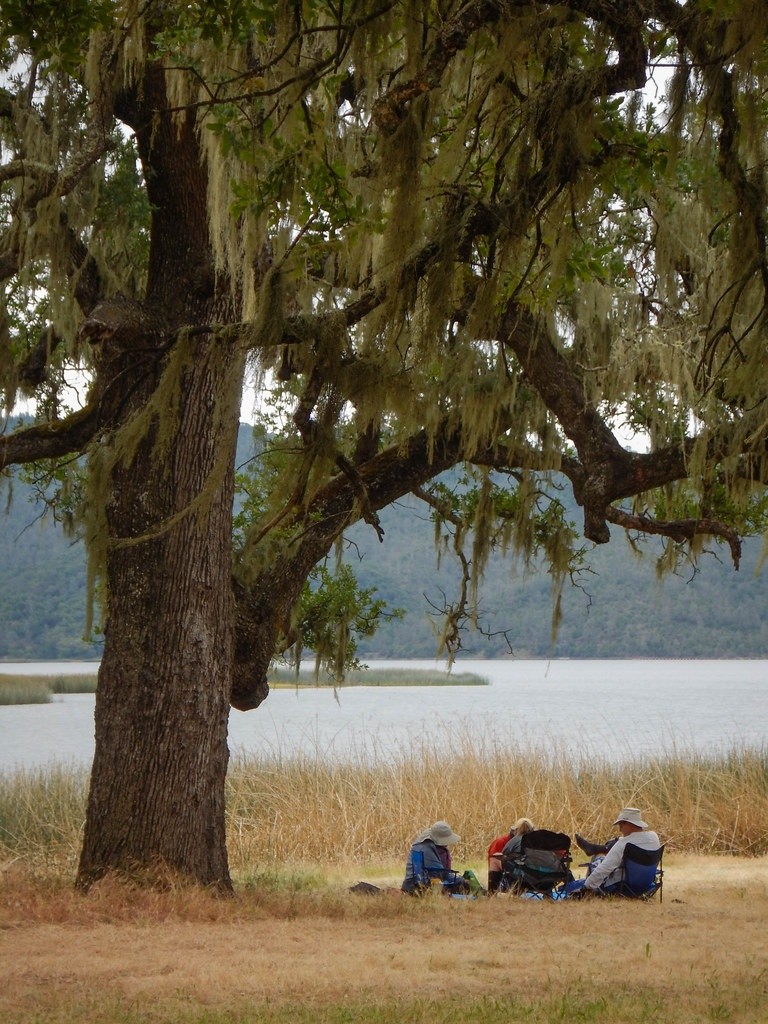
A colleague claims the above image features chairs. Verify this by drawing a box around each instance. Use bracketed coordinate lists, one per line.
[411, 849, 463, 898]
[490, 829, 572, 901]
[578, 842, 667, 904]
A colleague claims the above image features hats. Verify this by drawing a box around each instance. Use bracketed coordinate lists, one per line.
[417, 820, 461, 846]
[613, 808, 649, 828]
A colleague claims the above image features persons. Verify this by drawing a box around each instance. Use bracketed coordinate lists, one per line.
[502, 817, 553, 899]
[486, 822, 517, 894]
[575, 809, 659, 891]
[404, 819, 514, 900]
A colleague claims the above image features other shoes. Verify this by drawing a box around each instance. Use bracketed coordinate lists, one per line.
[575, 834, 594, 856]
